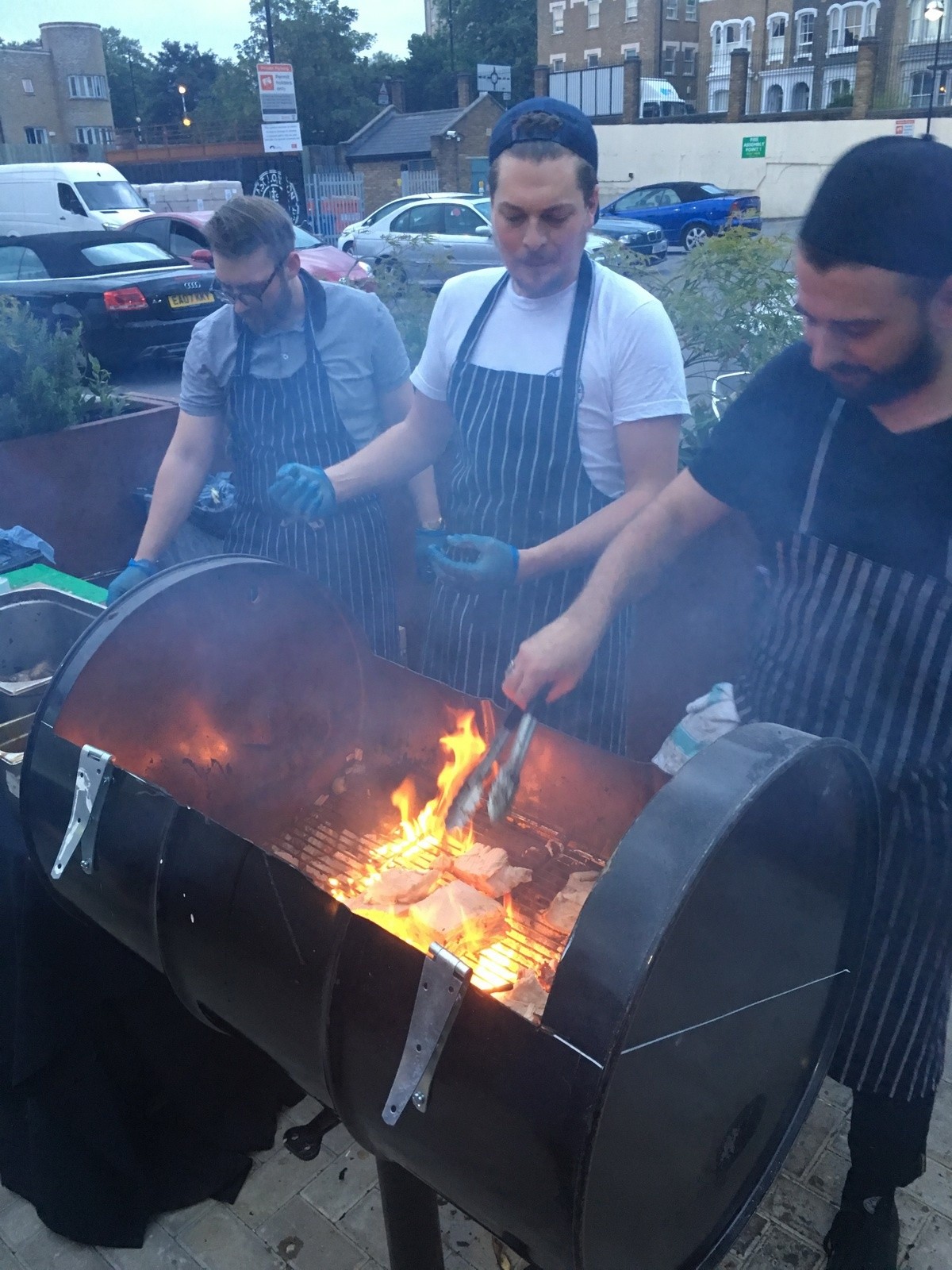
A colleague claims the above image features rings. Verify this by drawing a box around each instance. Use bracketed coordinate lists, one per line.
[511, 660, 514, 668]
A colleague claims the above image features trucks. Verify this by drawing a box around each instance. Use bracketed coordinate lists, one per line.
[638, 78, 687, 119]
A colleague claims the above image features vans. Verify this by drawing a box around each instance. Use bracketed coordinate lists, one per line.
[0, 162, 156, 236]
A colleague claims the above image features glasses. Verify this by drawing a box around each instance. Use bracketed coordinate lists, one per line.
[209, 259, 286, 309]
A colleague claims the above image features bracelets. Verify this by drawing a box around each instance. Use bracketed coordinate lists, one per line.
[419, 518, 444, 530]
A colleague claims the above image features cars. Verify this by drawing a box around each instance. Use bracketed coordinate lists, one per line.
[337, 192, 624, 299]
[116, 210, 378, 296]
[587, 214, 670, 267]
[597, 181, 763, 253]
[0, 233, 227, 387]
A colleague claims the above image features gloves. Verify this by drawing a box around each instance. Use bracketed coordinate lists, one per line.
[103, 558, 159, 607]
[271, 462, 339, 524]
[427, 532, 520, 594]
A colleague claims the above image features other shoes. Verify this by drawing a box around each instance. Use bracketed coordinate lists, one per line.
[823, 1168, 902, 1269]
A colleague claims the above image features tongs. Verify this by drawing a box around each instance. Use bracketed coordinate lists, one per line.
[443, 682, 554, 827]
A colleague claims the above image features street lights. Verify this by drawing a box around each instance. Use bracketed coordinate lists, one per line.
[136, 117, 142, 144]
[924, 0, 945, 137]
[178, 86, 187, 118]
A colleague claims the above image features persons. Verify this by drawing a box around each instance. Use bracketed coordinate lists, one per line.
[106, 193, 448, 665]
[501, 135, 952, 1270]
[268, 97, 691, 756]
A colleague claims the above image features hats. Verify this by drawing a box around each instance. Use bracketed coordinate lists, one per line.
[487, 96, 600, 224]
[800, 133, 952, 282]
